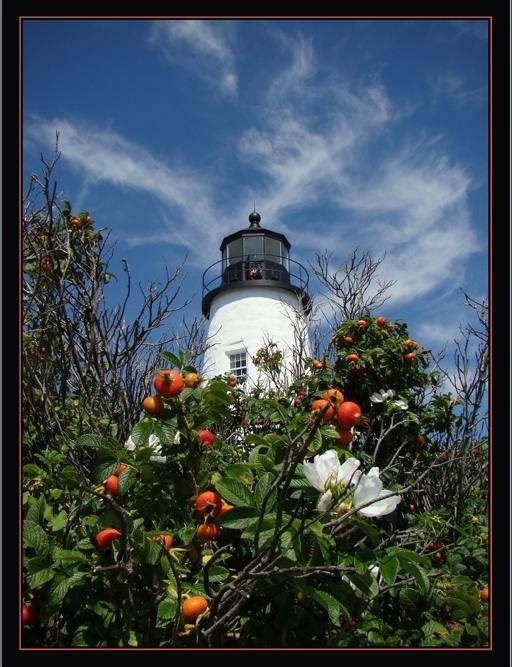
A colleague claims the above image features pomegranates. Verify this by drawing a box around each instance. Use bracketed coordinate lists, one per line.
[94, 317, 444, 620]
[23, 604, 30, 624]
[481, 588, 488, 602]
